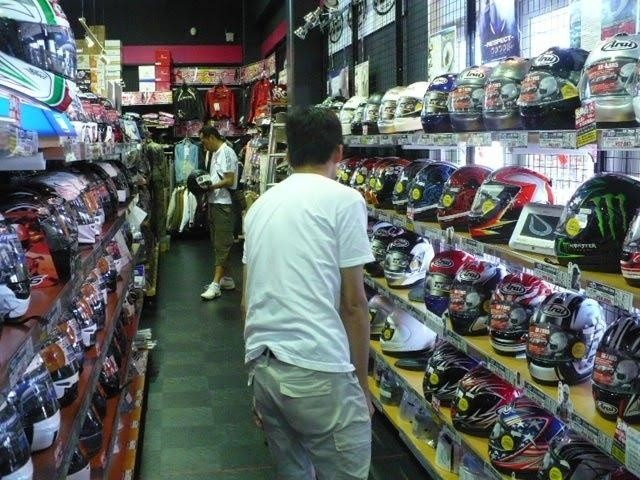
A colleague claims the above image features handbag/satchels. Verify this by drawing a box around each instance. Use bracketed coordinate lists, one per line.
[216, 171, 247, 213]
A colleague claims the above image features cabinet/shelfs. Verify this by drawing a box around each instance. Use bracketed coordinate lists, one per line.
[340, 126, 640, 476]
[0, 141, 160, 480]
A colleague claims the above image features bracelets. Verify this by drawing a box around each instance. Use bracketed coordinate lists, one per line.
[208, 185, 211, 192]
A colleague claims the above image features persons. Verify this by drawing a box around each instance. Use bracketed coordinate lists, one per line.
[197, 126, 238, 300]
[243, 108, 375, 480]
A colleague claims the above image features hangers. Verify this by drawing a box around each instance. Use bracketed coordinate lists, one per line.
[172, 130, 204, 145]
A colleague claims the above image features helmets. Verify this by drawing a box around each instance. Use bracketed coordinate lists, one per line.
[379, 309, 438, 357]
[186, 168, 213, 196]
[320, 96, 335, 108]
[269, 83, 288, 105]
[328, 95, 350, 119]
[406, 161, 459, 221]
[482, 55, 537, 130]
[459, 451, 491, 480]
[372, 361, 382, 388]
[1, 158, 145, 479]
[383, 233, 435, 289]
[554, 172, 640, 274]
[536, 428, 622, 480]
[488, 393, 567, 480]
[489, 272, 553, 356]
[374, 158, 413, 207]
[516, 46, 590, 129]
[434, 430, 467, 475]
[447, 65, 494, 132]
[339, 154, 370, 187]
[351, 97, 369, 135]
[412, 403, 442, 445]
[368, 155, 400, 208]
[577, 31, 640, 128]
[467, 165, 555, 243]
[436, 164, 493, 230]
[338, 95, 364, 136]
[447, 260, 512, 335]
[392, 158, 438, 215]
[365, 226, 408, 276]
[450, 363, 520, 438]
[525, 292, 607, 386]
[361, 92, 385, 135]
[368, 293, 400, 341]
[423, 249, 476, 318]
[590, 316, 640, 422]
[619, 206, 640, 288]
[1, 0, 79, 113]
[399, 390, 422, 423]
[349, 155, 383, 199]
[393, 80, 431, 133]
[422, 342, 478, 407]
[370, 220, 393, 237]
[379, 372, 404, 406]
[420, 73, 459, 133]
[377, 85, 409, 134]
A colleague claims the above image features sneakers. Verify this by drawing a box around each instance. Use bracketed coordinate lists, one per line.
[201, 282, 222, 300]
[203, 276, 236, 290]
